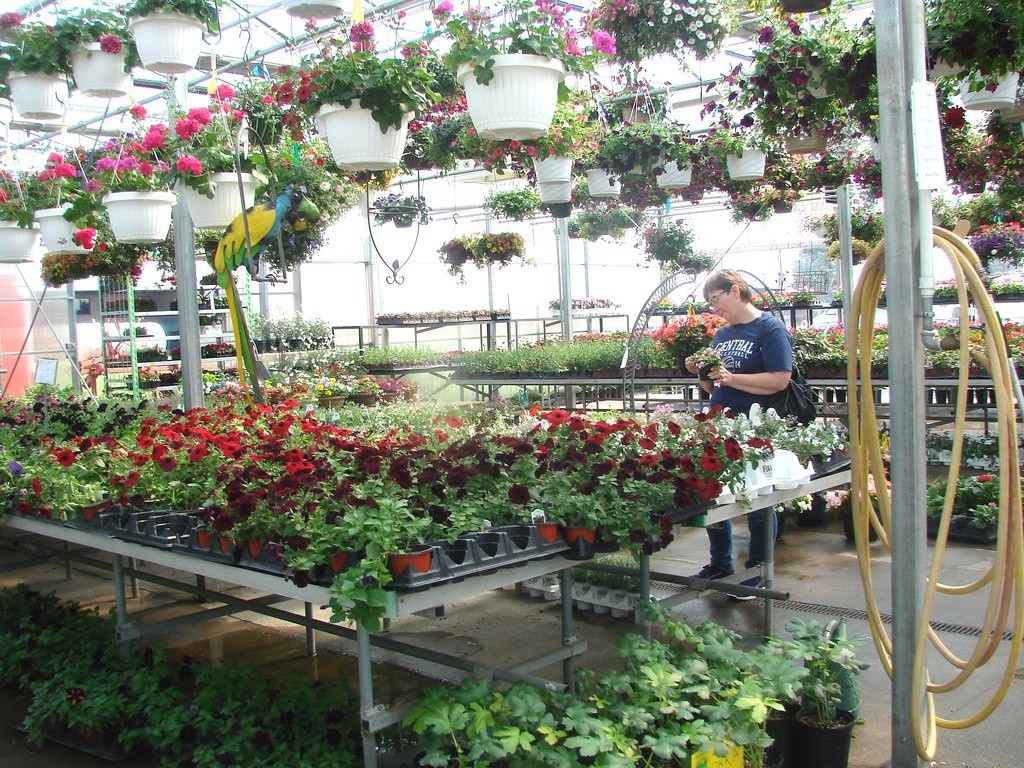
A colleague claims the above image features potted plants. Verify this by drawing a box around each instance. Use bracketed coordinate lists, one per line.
[0, 0, 1024, 768]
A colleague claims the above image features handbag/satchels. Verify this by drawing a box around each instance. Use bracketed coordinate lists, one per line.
[773, 361, 818, 427]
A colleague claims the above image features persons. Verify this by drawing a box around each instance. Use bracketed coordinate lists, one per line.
[685, 272, 795, 599]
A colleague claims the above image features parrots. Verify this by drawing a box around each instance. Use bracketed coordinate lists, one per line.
[213, 183, 321, 411]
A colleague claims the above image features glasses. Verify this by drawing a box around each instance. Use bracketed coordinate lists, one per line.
[710, 289, 729, 307]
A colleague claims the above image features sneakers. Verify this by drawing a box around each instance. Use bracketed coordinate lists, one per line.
[727, 561, 766, 601]
[689, 561, 735, 581]
